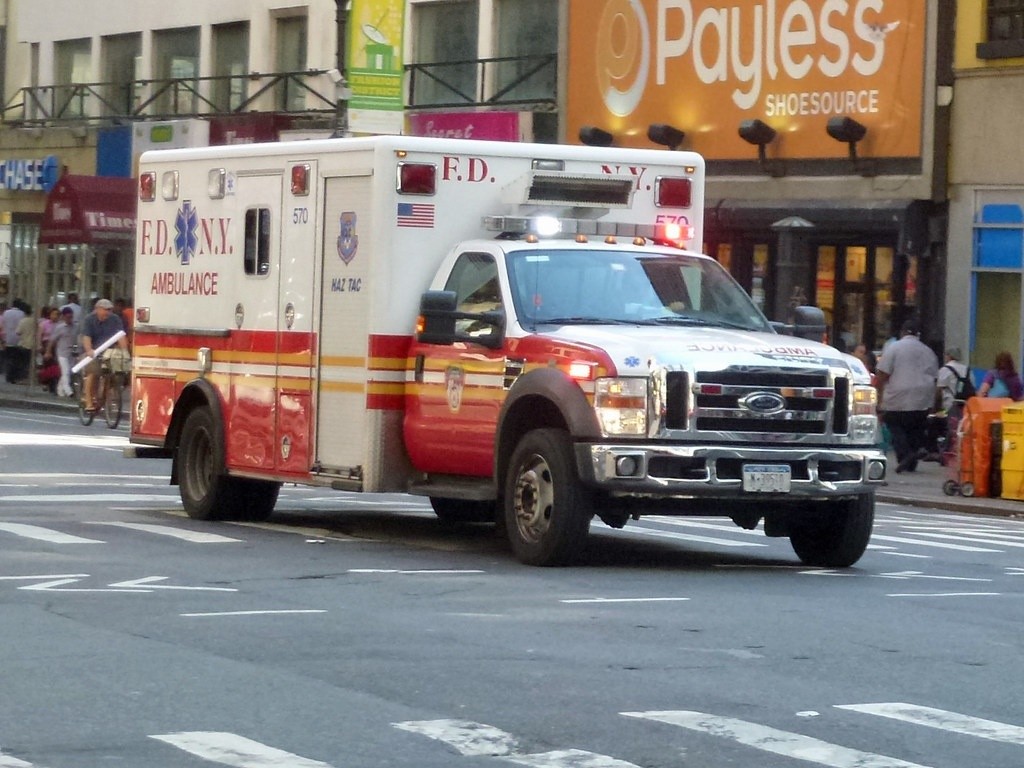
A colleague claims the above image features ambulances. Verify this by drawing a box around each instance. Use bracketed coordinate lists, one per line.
[125, 134, 890, 568]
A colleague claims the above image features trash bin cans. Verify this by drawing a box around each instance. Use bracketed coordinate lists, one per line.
[960, 397, 1012, 498]
[1001, 402, 1024, 500]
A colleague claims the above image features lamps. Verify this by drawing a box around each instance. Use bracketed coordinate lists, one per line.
[827, 115, 867, 174]
[738, 118, 777, 176]
[647, 123, 685, 150]
[578, 126, 613, 147]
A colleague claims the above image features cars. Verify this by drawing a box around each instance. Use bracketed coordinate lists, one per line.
[48, 291, 98, 310]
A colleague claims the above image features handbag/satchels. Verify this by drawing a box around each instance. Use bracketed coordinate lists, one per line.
[39, 360, 61, 380]
[987, 369, 1010, 397]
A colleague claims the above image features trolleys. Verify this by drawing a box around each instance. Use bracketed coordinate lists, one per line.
[943, 399, 975, 497]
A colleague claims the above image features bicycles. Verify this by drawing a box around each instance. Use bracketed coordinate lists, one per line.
[77, 354, 123, 429]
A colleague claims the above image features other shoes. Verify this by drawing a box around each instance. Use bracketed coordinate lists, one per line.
[916, 446, 939, 461]
[85, 407, 95, 412]
[68, 391, 73, 396]
[59, 393, 65, 397]
[894, 453, 919, 473]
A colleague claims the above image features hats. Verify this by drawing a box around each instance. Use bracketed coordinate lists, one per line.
[95, 299, 115, 310]
[945, 346, 962, 361]
[62, 307, 73, 314]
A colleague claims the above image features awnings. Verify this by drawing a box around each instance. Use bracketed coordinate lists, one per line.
[38, 175, 138, 243]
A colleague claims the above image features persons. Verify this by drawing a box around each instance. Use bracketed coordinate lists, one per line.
[0, 292, 134, 411]
[852, 318, 1024, 475]
[624, 265, 685, 315]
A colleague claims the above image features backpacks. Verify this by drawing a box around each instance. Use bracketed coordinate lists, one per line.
[942, 364, 977, 400]
